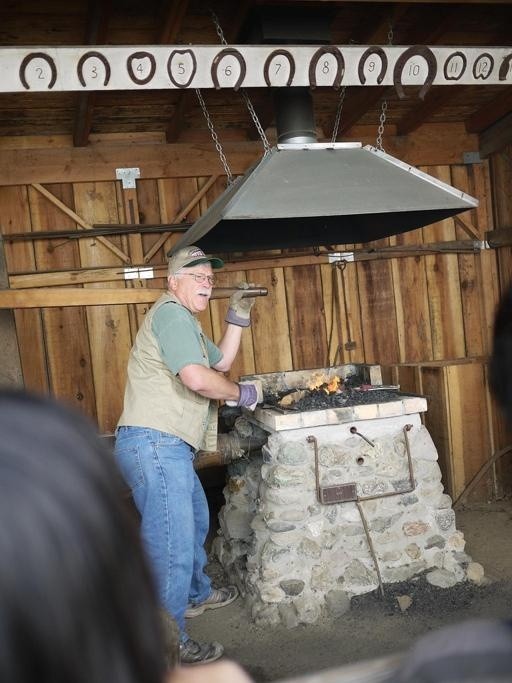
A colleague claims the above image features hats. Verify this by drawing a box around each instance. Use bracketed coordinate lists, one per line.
[165, 245, 224, 277]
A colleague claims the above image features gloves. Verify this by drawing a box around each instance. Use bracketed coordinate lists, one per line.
[225, 380, 264, 413]
[225, 282, 262, 328]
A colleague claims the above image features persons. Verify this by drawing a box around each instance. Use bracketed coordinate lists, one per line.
[367, 283, 510, 683]
[1, 382, 252, 680]
[116, 243, 265, 665]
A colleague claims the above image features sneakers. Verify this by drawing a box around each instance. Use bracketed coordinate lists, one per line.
[185, 585, 240, 618]
[178, 637, 225, 666]
